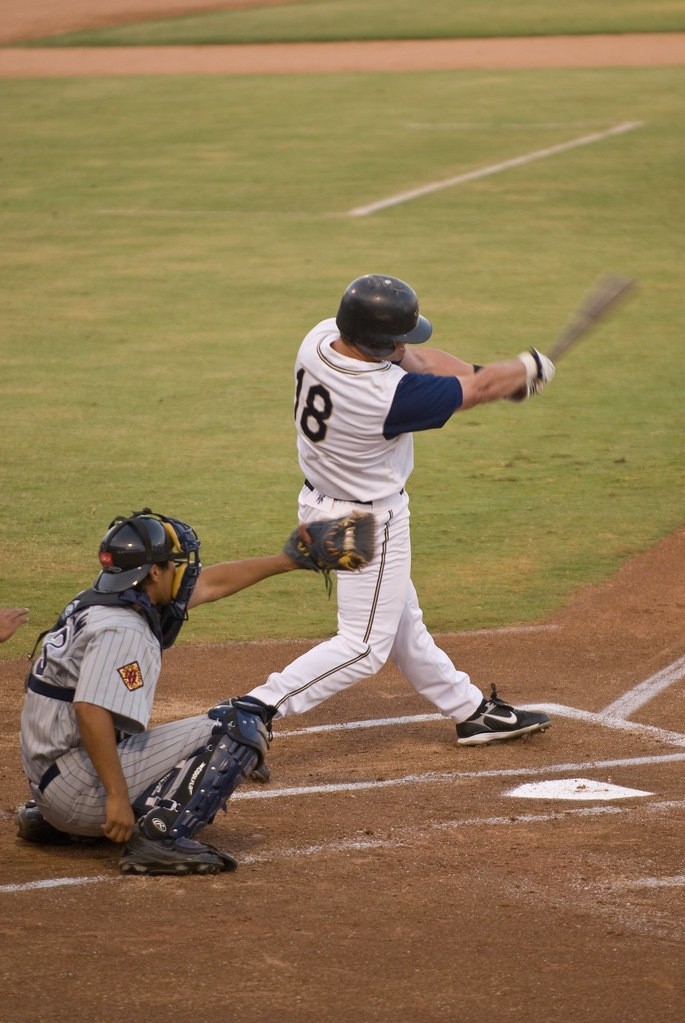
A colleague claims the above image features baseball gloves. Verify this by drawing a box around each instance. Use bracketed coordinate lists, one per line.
[283, 507, 378, 602]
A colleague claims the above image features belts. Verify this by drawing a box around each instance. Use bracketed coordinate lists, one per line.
[304, 478, 404, 505]
[28, 728, 130, 794]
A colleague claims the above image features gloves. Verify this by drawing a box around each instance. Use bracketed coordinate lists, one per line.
[506, 346, 556, 403]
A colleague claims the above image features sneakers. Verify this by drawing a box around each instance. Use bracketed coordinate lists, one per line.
[119, 833, 224, 876]
[14, 799, 50, 845]
[456, 683, 551, 746]
[208, 696, 278, 783]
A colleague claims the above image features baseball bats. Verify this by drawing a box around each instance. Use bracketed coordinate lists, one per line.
[510, 280, 630, 402]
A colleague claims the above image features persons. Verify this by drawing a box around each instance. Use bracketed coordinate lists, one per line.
[209, 272, 558, 783]
[17, 509, 378, 871]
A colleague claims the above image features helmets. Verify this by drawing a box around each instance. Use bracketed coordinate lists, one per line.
[336, 274, 433, 359]
[92, 515, 175, 594]
